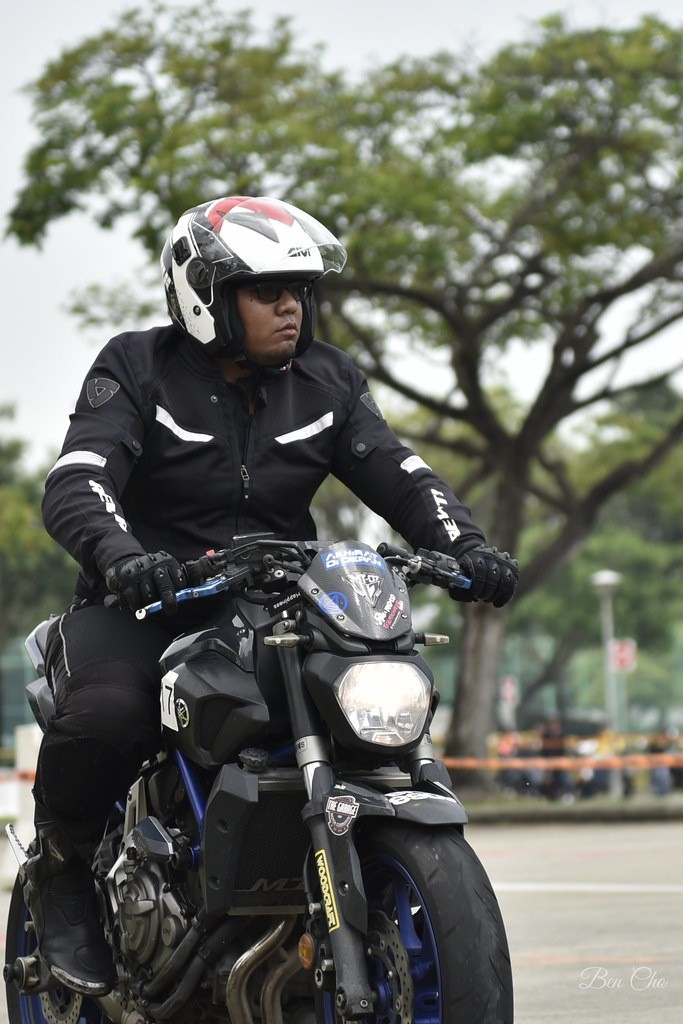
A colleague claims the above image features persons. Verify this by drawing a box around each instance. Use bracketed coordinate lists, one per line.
[24, 196, 518, 996]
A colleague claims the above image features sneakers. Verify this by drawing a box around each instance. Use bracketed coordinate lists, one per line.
[20, 854, 118, 999]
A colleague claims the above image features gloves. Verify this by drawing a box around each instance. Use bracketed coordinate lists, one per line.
[106, 550, 186, 617]
[448, 544, 520, 607]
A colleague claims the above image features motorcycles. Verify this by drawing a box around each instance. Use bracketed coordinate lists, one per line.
[0, 531, 515, 1022]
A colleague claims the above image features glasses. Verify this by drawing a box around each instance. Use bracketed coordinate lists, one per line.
[242, 279, 312, 303]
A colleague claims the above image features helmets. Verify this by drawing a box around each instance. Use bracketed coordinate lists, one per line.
[161, 196, 348, 353]
